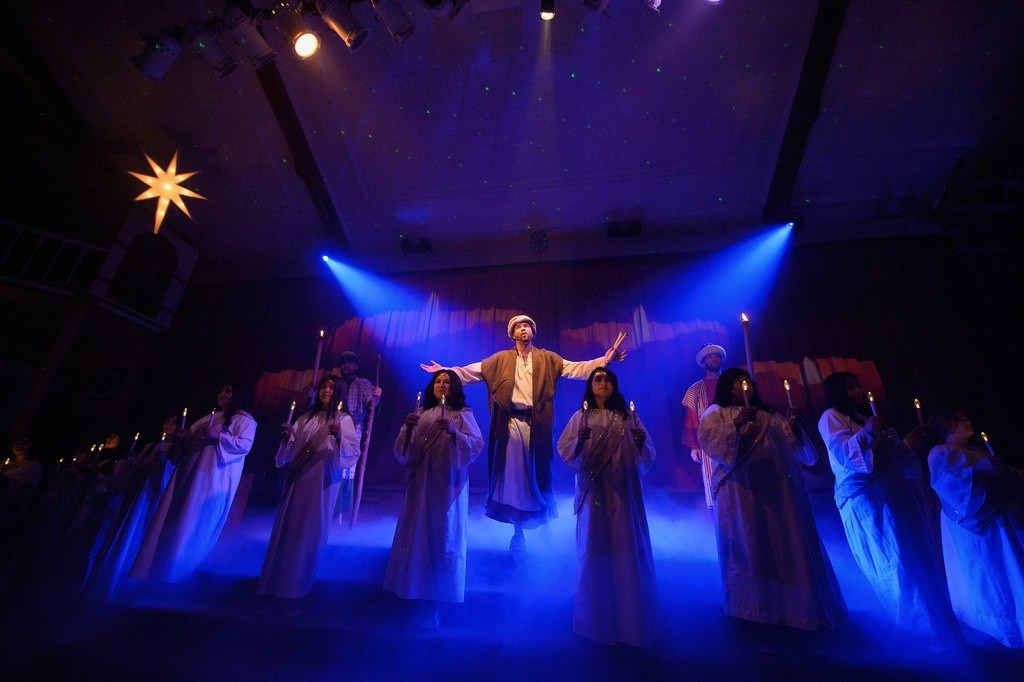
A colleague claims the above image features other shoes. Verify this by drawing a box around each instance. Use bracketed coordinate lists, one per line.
[420, 615, 439, 630]
[757, 641, 778, 655]
[803, 646, 829, 661]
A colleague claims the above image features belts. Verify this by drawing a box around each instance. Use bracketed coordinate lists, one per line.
[511, 408, 531, 417]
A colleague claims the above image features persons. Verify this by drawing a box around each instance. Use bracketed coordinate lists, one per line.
[255, 350, 383, 601]
[681, 342, 728, 508]
[380, 370, 485, 630]
[0, 381, 258, 602]
[818, 372, 967, 653]
[696, 367, 852, 630]
[926, 409, 1024, 648]
[416, 315, 631, 526]
[555, 367, 665, 648]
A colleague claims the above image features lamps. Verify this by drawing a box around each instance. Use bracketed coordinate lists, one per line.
[130, 0, 660, 89]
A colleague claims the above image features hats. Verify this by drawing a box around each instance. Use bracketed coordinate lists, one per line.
[508, 314, 537, 342]
[696, 343, 727, 368]
[338, 351, 358, 365]
